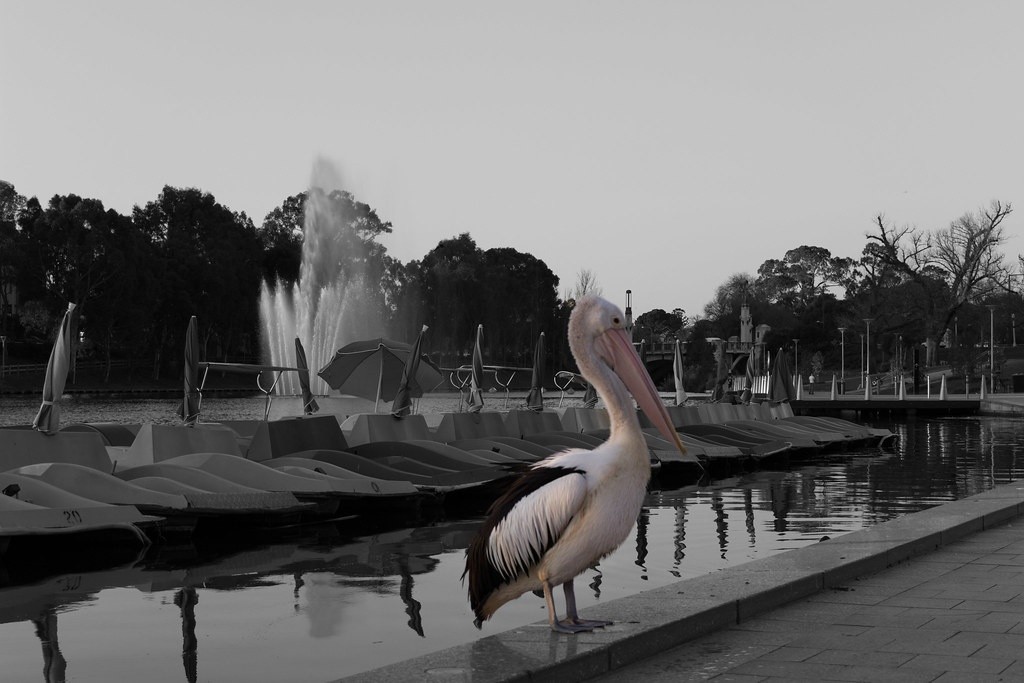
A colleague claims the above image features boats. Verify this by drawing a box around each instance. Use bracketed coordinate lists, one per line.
[1, 396, 896, 569]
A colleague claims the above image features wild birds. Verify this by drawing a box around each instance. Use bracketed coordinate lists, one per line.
[459, 293, 688, 635]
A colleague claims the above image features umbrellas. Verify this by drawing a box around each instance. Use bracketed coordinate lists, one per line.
[316, 337, 446, 415]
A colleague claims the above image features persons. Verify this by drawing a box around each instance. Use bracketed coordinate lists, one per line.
[808, 373, 815, 395]
[727, 375, 733, 392]
[871, 376, 879, 386]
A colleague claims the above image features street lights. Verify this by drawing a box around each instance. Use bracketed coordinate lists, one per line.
[1011, 313, 1018, 348]
[862, 318, 877, 396]
[985, 304, 999, 396]
[838, 327, 847, 395]
[893, 333, 902, 397]
[792, 339, 799, 392]
